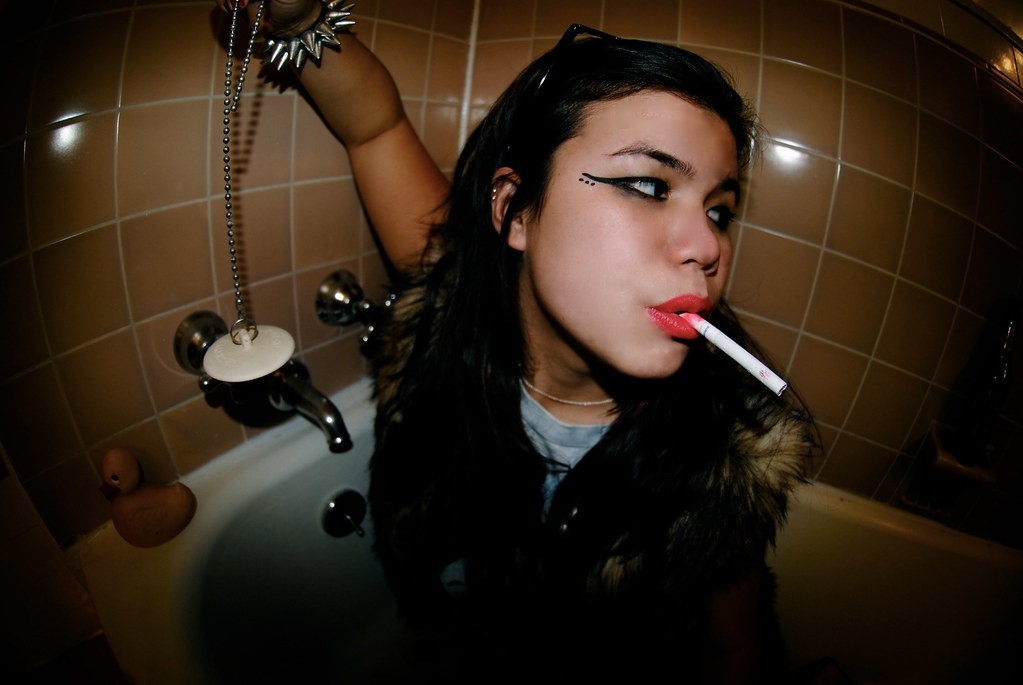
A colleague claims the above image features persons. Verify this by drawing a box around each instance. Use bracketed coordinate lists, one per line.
[216, 0, 827, 685]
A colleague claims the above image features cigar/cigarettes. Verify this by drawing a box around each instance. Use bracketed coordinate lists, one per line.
[678, 313, 787, 397]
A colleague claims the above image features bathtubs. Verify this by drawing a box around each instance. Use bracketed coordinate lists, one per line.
[70, 376, 1023, 685]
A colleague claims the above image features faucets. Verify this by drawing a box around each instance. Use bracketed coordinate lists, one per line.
[268, 353, 354, 453]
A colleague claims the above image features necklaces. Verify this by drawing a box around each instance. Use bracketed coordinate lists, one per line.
[522, 377, 614, 406]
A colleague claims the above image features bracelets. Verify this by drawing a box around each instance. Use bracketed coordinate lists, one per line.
[262, 0, 356, 72]
